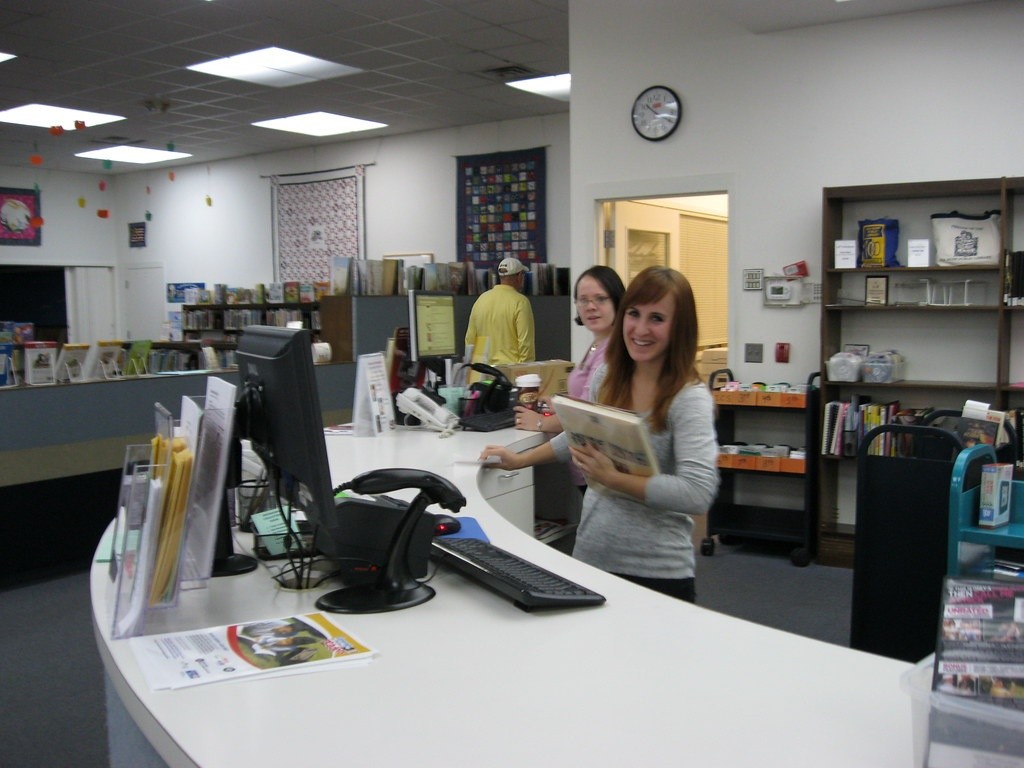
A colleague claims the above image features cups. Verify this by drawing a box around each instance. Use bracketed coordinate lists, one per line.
[515, 374, 540, 411]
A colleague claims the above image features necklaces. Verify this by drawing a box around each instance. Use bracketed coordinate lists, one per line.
[592, 342, 600, 349]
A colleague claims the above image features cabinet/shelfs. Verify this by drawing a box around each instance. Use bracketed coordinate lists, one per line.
[849, 409, 1019, 664]
[699, 369, 822, 568]
[182, 301, 324, 371]
[946, 442, 1024, 582]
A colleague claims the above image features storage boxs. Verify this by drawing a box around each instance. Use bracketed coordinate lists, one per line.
[823, 352, 877, 382]
[979, 462, 1013, 530]
[860, 350, 906, 384]
[490, 358, 574, 406]
[899, 650, 1024, 768]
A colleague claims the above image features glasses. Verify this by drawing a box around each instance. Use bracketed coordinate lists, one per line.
[574, 295, 612, 305]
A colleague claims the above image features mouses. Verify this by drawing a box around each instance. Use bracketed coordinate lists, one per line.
[433, 513, 460, 535]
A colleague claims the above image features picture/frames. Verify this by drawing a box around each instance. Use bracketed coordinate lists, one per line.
[842, 344, 870, 358]
[0, 187, 41, 247]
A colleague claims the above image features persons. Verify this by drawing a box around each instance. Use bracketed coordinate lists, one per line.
[513, 266, 626, 498]
[478, 264, 722, 605]
[464, 257, 536, 364]
[244, 619, 318, 654]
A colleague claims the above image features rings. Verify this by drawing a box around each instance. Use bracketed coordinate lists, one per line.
[516, 418, 520, 423]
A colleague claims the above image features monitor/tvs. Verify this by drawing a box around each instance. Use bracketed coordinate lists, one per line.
[209, 324, 335, 578]
[410, 290, 459, 379]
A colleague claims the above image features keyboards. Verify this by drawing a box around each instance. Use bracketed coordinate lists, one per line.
[458, 407, 516, 431]
[430, 538, 606, 613]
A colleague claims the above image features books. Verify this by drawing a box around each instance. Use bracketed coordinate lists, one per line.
[550, 391, 659, 504]
[222, 308, 262, 331]
[125, 339, 151, 375]
[149, 346, 237, 373]
[1003, 249, 1024, 306]
[185, 281, 330, 303]
[311, 311, 322, 330]
[57, 343, 89, 379]
[23, 341, 57, 383]
[353, 257, 569, 296]
[406, 288, 459, 362]
[182, 309, 216, 330]
[92, 340, 122, 378]
[265, 307, 302, 327]
[821, 394, 1017, 458]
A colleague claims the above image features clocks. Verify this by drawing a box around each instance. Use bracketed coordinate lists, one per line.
[631, 86, 682, 142]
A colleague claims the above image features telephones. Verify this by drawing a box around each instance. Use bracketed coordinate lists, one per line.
[396, 388, 460, 432]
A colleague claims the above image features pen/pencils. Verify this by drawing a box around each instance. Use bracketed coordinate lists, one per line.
[463, 391, 480, 417]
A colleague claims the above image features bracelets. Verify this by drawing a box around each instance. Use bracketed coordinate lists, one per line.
[536, 417, 543, 431]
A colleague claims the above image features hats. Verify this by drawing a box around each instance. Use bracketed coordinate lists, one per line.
[497, 258, 530, 276]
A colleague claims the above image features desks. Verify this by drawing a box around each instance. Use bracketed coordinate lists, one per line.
[815, 177, 1024, 570]
